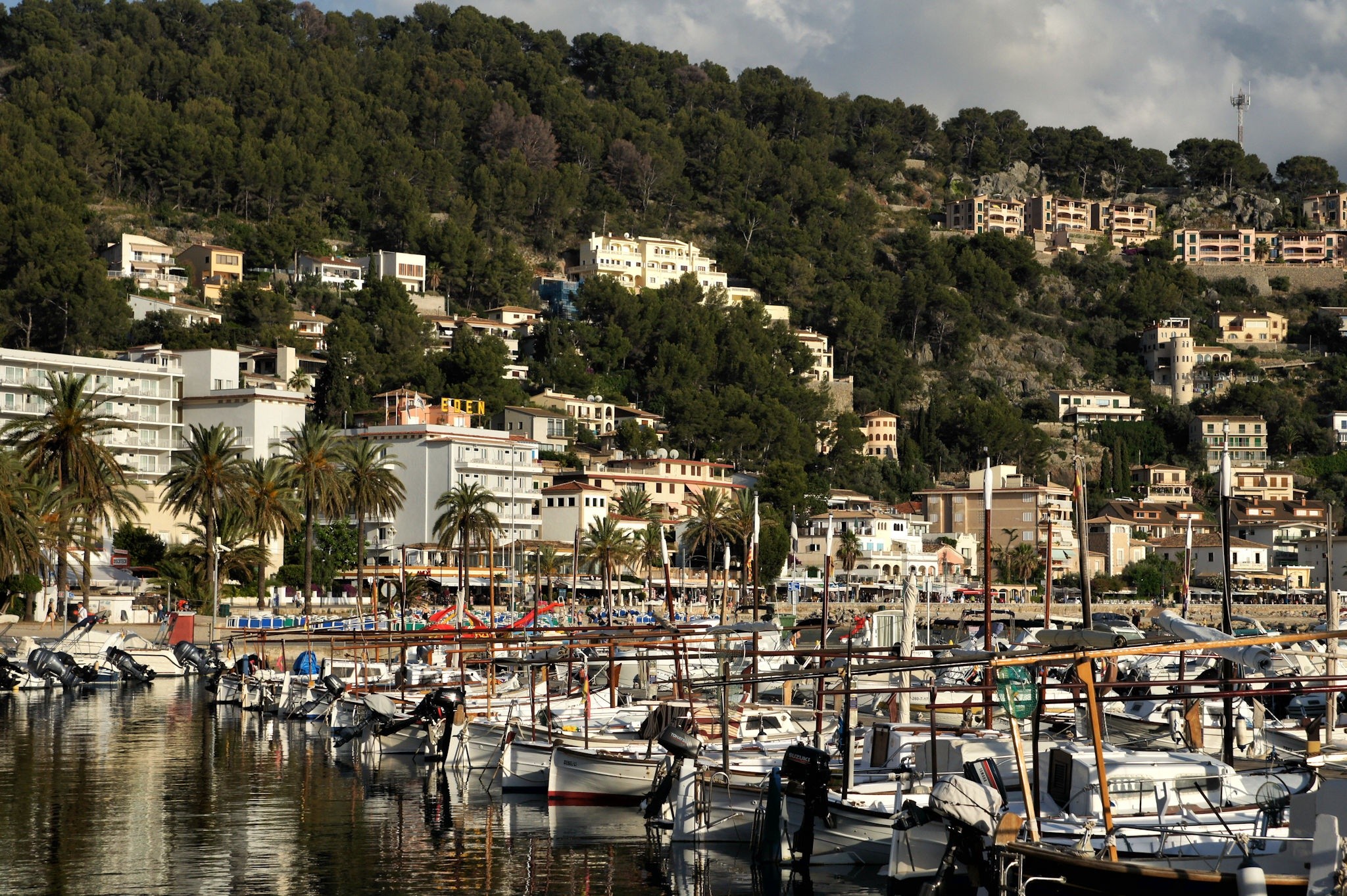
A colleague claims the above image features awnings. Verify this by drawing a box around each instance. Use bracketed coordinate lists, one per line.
[428, 576, 519, 588]
[649, 579, 764, 590]
[953, 590, 997, 594]
[555, 579, 644, 590]
[801, 584, 904, 592]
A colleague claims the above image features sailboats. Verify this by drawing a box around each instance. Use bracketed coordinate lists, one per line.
[0, 415, 1347, 896]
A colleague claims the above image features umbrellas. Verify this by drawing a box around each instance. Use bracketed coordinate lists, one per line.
[1266, 587, 1309, 595]
[1052, 587, 1081, 594]
[1191, 591, 1240, 596]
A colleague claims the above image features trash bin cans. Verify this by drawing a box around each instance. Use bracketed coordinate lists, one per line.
[169, 612, 197, 645]
[1140, 610, 1145, 617]
[220, 604, 229, 617]
[775, 614, 796, 641]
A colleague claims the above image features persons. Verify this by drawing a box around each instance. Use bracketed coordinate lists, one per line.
[156, 598, 189, 623]
[1015, 594, 1024, 604]
[1191, 595, 1324, 605]
[1131, 608, 1140, 628]
[77, 602, 89, 632]
[550, 593, 636, 633]
[1210, 609, 1213, 622]
[654, 590, 768, 618]
[293, 592, 304, 608]
[1153, 594, 1161, 608]
[411, 588, 476, 623]
[917, 592, 984, 604]
[40, 598, 58, 632]
[1054, 595, 1101, 603]
[811, 590, 894, 603]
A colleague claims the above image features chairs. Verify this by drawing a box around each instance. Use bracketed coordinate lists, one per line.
[226, 610, 729, 630]
